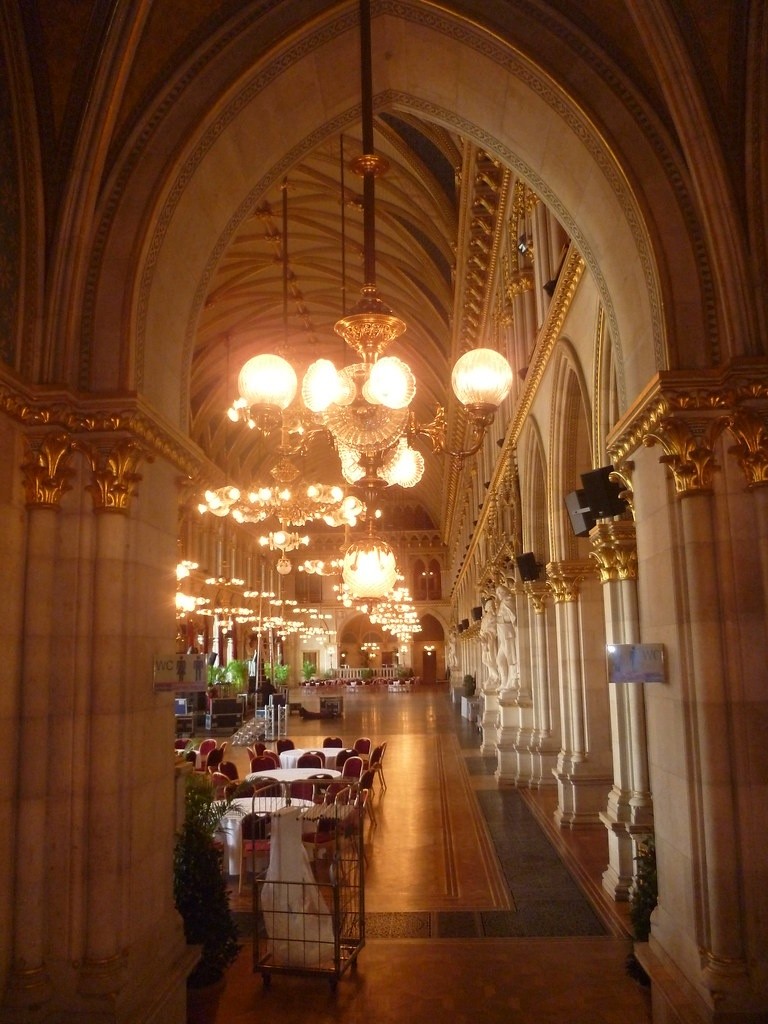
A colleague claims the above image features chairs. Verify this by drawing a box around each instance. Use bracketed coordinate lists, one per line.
[174, 734, 391, 892]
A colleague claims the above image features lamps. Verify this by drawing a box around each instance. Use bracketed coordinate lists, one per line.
[226, 2, 514, 491]
[199, 175, 363, 575]
[343, 501, 405, 615]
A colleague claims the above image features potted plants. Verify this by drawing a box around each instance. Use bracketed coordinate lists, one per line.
[174, 774, 244, 1024]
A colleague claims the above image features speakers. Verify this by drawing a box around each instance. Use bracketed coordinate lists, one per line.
[565, 465, 627, 537]
[471, 607, 482, 622]
[516, 552, 539, 582]
[457, 619, 470, 633]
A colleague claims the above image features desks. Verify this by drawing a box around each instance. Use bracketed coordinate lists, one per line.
[279, 748, 348, 772]
[202, 797, 313, 875]
[244, 768, 341, 795]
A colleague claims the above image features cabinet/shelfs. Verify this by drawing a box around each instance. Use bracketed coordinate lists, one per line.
[252, 780, 366, 993]
